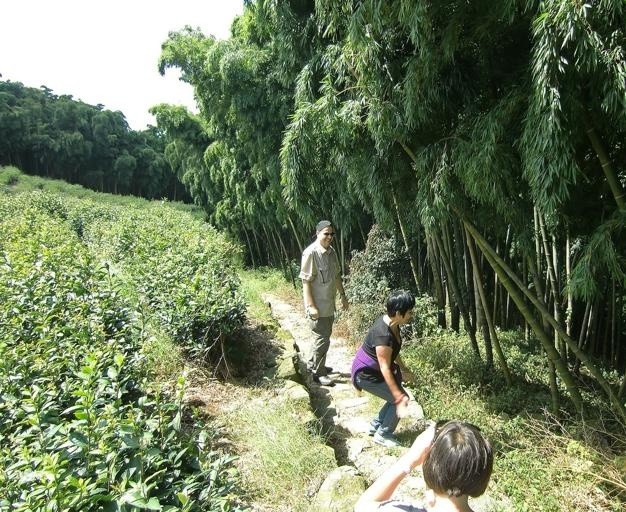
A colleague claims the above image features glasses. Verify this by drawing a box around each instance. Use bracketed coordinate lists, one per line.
[435, 419, 481, 436]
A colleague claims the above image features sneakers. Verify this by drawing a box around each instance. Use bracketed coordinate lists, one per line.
[313, 372, 335, 387]
[368, 424, 400, 449]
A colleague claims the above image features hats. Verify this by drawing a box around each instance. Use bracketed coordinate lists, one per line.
[315, 220, 334, 232]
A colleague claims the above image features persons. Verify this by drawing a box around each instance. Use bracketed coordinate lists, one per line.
[353, 418, 495, 512]
[351, 289, 417, 448]
[298, 219, 350, 387]
